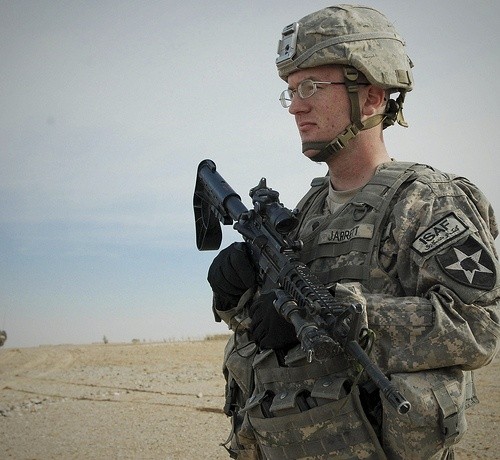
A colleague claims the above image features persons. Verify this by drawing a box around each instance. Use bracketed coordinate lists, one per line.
[208, 5, 500, 460]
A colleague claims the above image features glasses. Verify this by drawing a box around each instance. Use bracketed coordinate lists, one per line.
[279, 79, 387, 108]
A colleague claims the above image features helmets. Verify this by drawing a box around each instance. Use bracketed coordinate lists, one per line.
[275, 4, 414, 93]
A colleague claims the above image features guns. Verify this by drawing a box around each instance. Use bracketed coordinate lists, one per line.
[193, 158, 412, 415]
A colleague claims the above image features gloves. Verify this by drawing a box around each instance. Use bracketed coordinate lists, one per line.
[248, 291, 300, 349]
[208, 242, 257, 311]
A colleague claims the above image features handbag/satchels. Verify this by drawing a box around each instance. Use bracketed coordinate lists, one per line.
[249, 393, 387, 460]
